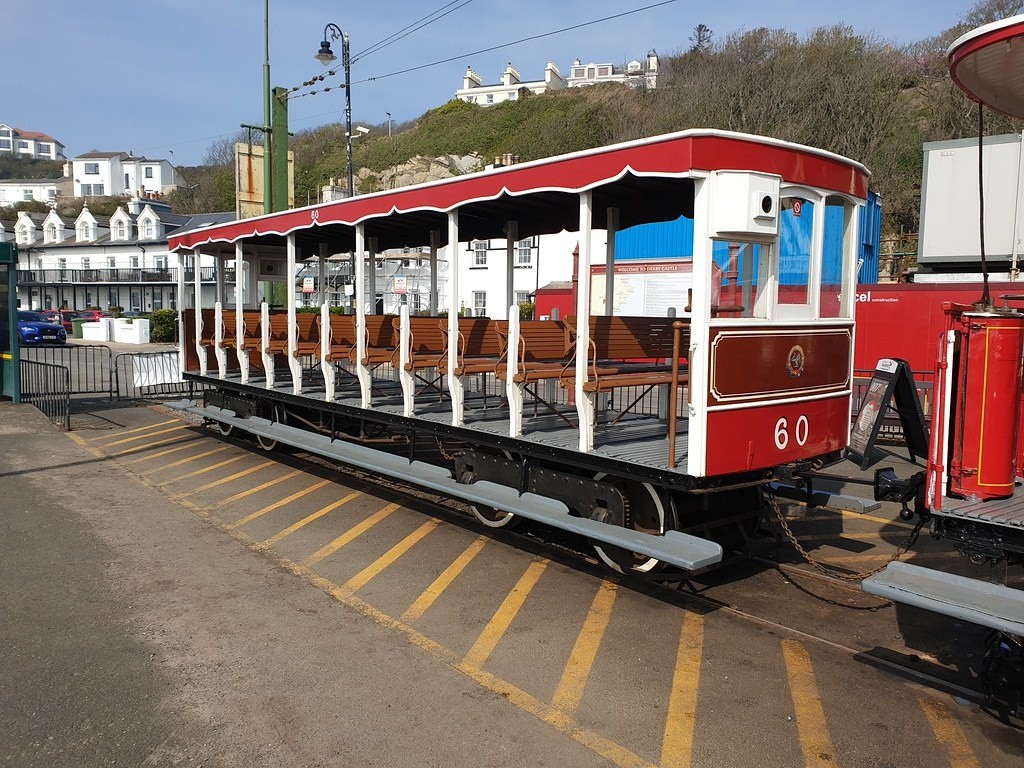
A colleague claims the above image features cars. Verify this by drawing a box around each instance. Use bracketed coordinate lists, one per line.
[16, 310, 66, 345]
[122, 312, 152, 318]
[40, 308, 80, 333]
[80, 311, 112, 323]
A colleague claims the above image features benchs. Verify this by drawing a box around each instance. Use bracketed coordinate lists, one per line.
[436, 319, 538, 416]
[256, 313, 323, 386]
[232, 312, 293, 378]
[282, 313, 361, 386]
[494, 321, 660, 428]
[313, 316, 389, 397]
[349, 315, 444, 403]
[391, 318, 492, 409]
[209, 310, 265, 374]
[559, 315, 690, 439]
[192, 310, 258, 369]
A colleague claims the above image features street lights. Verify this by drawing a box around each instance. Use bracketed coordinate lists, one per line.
[315, 21, 356, 315]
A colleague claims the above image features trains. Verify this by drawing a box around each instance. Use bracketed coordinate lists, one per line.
[161, 12, 1024, 708]
